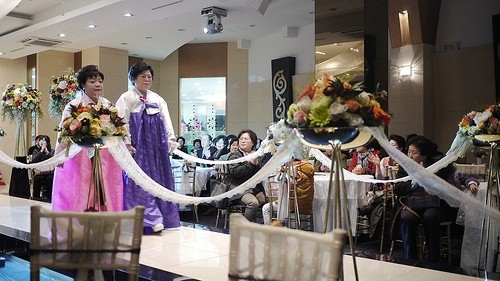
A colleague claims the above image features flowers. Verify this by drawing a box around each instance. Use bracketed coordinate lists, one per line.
[48, 68, 80, 116]
[55, 102, 127, 143]
[266, 119, 294, 144]
[2, 82, 45, 126]
[286, 72, 390, 129]
[457, 104, 500, 136]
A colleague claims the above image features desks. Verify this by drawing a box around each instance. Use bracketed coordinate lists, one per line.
[265, 173, 373, 236]
[174, 166, 207, 222]
[459, 181, 500, 273]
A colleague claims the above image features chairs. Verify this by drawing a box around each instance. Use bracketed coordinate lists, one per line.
[184, 157, 452, 271]
[227, 213, 347, 281]
[30, 204, 145, 281]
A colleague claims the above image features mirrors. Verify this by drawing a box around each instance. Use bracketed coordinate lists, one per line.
[178, 77, 227, 146]
[315, 0, 365, 89]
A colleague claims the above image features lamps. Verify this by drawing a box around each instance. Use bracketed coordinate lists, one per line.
[201, 7, 227, 34]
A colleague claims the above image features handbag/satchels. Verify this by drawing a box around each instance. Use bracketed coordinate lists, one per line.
[405, 190, 441, 212]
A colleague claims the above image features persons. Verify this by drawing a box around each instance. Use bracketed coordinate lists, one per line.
[116, 63, 181, 233]
[29, 130, 480, 271]
[51, 65, 128, 230]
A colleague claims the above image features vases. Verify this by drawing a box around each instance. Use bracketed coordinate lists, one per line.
[294, 128, 372, 148]
[69, 136, 104, 147]
[473, 135, 500, 148]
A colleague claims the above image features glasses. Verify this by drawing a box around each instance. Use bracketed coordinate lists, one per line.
[238, 137, 252, 143]
[137, 74, 152, 79]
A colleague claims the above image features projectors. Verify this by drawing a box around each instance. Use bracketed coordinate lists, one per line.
[200, 7, 228, 34]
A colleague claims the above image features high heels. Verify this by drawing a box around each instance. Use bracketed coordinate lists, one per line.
[201, 209, 217, 217]
